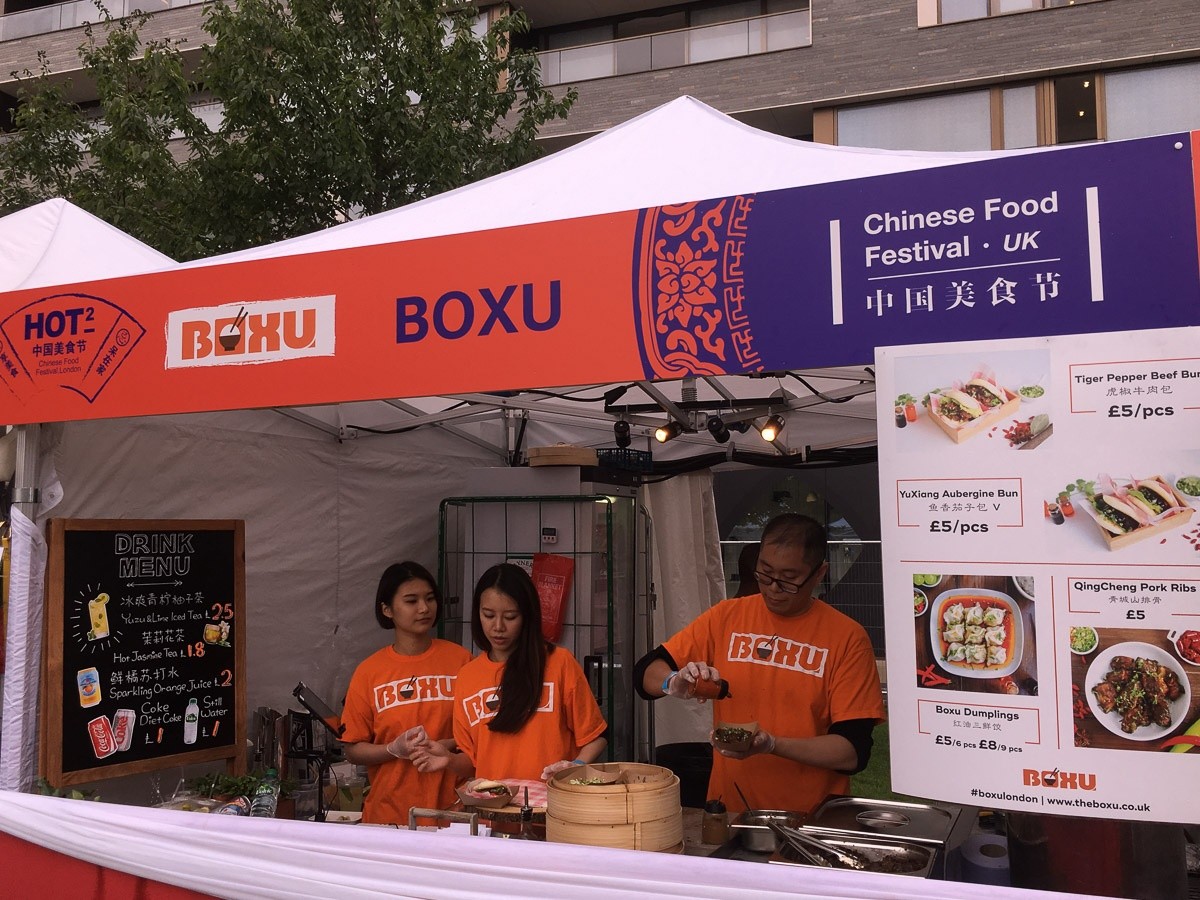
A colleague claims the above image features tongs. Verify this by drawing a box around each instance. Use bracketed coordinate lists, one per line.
[768, 820, 867, 869]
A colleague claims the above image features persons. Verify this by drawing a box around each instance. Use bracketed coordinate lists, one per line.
[341, 561, 476, 826]
[633, 513, 884, 813]
[406, 563, 608, 805]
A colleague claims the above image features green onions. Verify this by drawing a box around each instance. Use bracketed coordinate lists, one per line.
[569, 776, 602, 785]
[1070, 626, 1095, 652]
[1020, 385, 1043, 398]
[1176, 476, 1200, 496]
[1112, 657, 1159, 723]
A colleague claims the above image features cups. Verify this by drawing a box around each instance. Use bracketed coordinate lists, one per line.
[294, 772, 367, 820]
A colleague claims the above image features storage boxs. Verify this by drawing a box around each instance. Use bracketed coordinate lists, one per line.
[526, 445, 600, 466]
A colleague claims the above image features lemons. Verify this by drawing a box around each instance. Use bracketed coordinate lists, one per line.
[913, 574, 937, 585]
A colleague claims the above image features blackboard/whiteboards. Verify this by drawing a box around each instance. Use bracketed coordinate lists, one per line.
[43, 519, 247, 788]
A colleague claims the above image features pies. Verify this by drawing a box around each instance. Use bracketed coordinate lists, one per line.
[937, 379, 1008, 424]
[1092, 479, 1179, 534]
[472, 780, 508, 796]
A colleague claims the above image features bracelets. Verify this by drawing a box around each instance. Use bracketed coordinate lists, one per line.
[573, 760, 586, 764]
[662, 671, 677, 694]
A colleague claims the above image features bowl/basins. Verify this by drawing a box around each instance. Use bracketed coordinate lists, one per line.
[1071, 627, 1200, 741]
[930, 588, 1024, 679]
[731, 808, 804, 852]
[1012, 576, 1034, 602]
[1173, 473, 1200, 502]
[1018, 383, 1047, 404]
[308, 810, 363, 825]
[913, 574, 943, 618]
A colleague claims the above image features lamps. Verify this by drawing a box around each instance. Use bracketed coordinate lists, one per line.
[707, 398, 759, 444]
[613, 411, 630, 448]
[761, 404, 785, 441]
[655, 411, 683, 443]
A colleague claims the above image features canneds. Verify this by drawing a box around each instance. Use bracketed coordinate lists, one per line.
[88, 709, 136, 758]
[77, 667, 101, 708]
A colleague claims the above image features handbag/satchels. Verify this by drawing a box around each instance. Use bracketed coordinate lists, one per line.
[531, 553, 575, 645]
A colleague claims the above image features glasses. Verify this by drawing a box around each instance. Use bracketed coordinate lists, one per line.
[752, 561, 823, 595]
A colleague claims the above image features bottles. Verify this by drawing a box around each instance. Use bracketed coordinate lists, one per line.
[1000, 673, 1038, 696]
[687, 673, 730, 700]
[250, 768, 281, 818]
[1048, 495, 1074, 526]
[513, 806, 541, 841]
[702, 799, 731, 845]
[895, 400, 917, 429]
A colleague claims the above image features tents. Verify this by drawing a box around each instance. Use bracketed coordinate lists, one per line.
[0, 96, 1200, 900]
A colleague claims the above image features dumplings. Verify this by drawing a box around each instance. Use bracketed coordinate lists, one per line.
[943, 601, 1007, 666]
